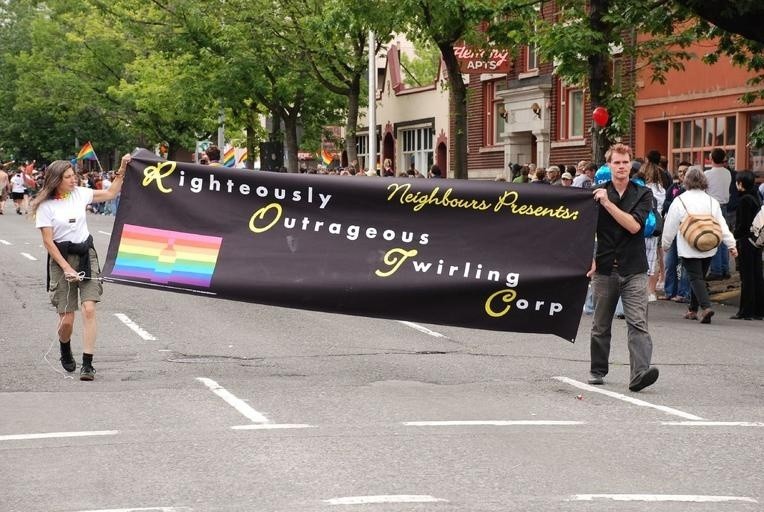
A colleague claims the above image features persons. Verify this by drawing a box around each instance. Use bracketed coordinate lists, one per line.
[508, 147, 764, 323]
[587, 144, 659, 392]
[0, 142, 225, 217]
[272, 159, 445, 179]
[30, 152, 132, 381]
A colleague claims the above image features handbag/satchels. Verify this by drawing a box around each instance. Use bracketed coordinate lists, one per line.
[651, 208, 663, 236]
[678, 213, 723, 253]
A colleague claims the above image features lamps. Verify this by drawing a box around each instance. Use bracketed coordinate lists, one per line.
[530, 102, 542, 120]
[497, 105, 509, 123]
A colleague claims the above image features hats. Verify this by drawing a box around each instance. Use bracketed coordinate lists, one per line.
[561, 172, 573, 179]
[546, 165, 560, 171]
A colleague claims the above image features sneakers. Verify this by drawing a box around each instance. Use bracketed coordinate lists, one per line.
[0, 206, 30, 217]
[647, 291, 657, 302]
[703, 272, 732, 281]
[657, 292, 686, 303]
[79, 363, 96, 381]
[587, 369, 604, 385]
[683, 309, 698, 320]
[86, 205, 117, 218]
[628, 367, 659, 392]
[60, 350, 76, 372]
[699, 308, 715, 324]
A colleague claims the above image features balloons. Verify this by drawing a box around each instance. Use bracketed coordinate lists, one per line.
[593, 107, 610, 129]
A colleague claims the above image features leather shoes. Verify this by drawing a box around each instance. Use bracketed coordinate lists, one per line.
[729, 310, 763, 321]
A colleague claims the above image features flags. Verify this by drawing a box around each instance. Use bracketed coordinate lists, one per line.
[222, 148, 235, 168]
[321, 149, 334, 168]
